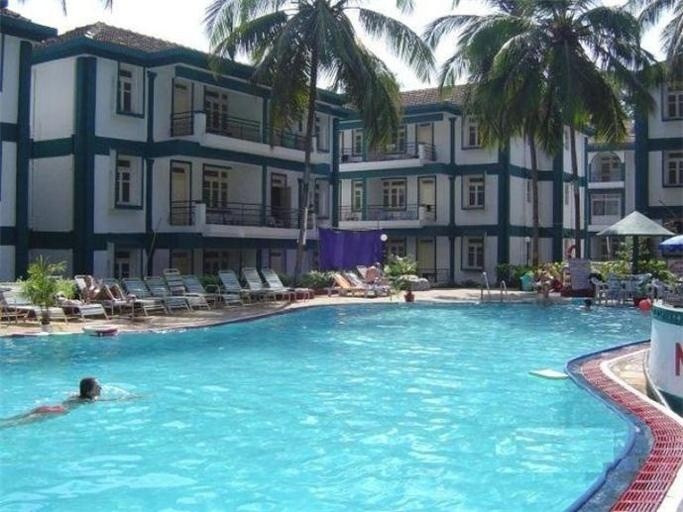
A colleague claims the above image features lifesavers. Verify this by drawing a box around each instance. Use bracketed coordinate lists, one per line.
[81, 323, 117, 337]
[568, 244, 580, 259]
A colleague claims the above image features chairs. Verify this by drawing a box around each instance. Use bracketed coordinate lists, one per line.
[2, 281, 69, 326]
[591, 271, 671, 308]
[163, 268, 211, 312]
[241, 266, 296, 303]
[261, 267, 314, 301]
[183, 274, 244, 309]
[324, 262, 392, 296]
[144, 278, 190, 314]
[73, 275, 141, 322]
[217, 270, 274, 305]
[123, 278, 171, 316]
[48, 274, 109, 325]
[101, 277, 161, 316]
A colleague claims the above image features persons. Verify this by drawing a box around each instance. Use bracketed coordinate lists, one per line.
[366, 262, 387, 285]
[404, 288, 414, 301]
[55, 294, 90, 306]
[83, 275, 128, 302]
[580, 300, 593, 312]
[0, 378, 142, 427]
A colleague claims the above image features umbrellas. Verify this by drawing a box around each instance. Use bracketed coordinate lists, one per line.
[659, 234, 683, 252]
[596, 211, 676, 274]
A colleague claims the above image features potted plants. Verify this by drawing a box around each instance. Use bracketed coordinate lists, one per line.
[388, 253, 418, 303]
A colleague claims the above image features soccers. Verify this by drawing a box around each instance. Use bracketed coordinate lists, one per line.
[639, 299, 650, 308]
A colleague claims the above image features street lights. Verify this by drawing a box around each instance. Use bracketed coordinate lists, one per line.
[380, 234, 388, 264]
[524, 236, 531, 267]
